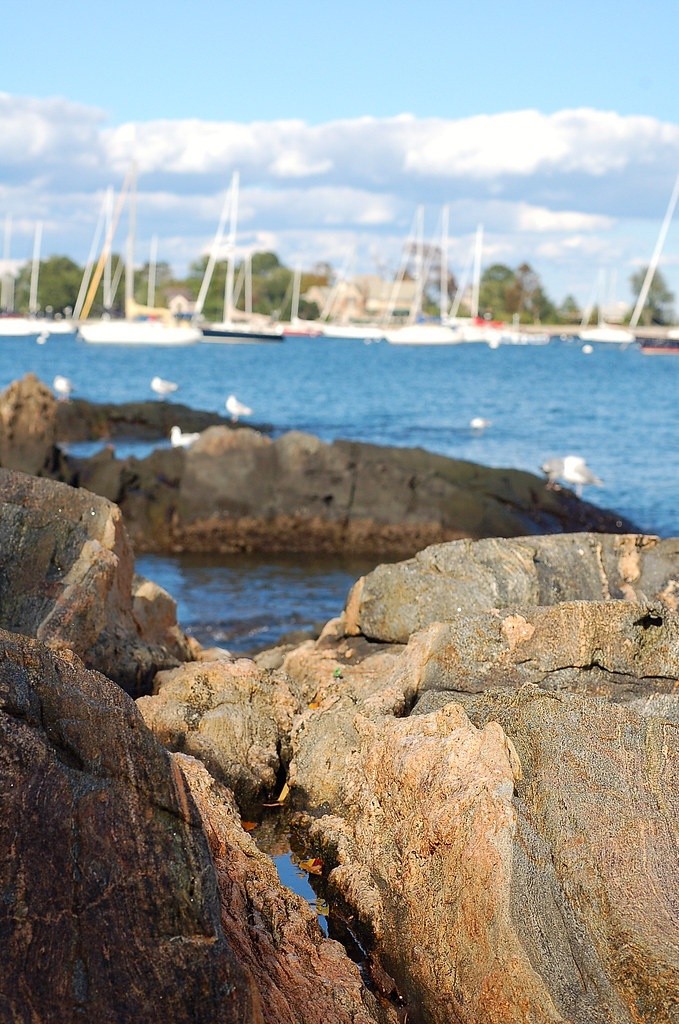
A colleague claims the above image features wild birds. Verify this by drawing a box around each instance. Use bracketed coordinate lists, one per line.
[225, 394, 253, 424]
[170, 425, 201, 447]
[52, 374, 76, 401]
[150, 375, 178, 403]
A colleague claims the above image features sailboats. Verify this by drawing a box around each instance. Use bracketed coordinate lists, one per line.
[0, 164, 678, 358]
